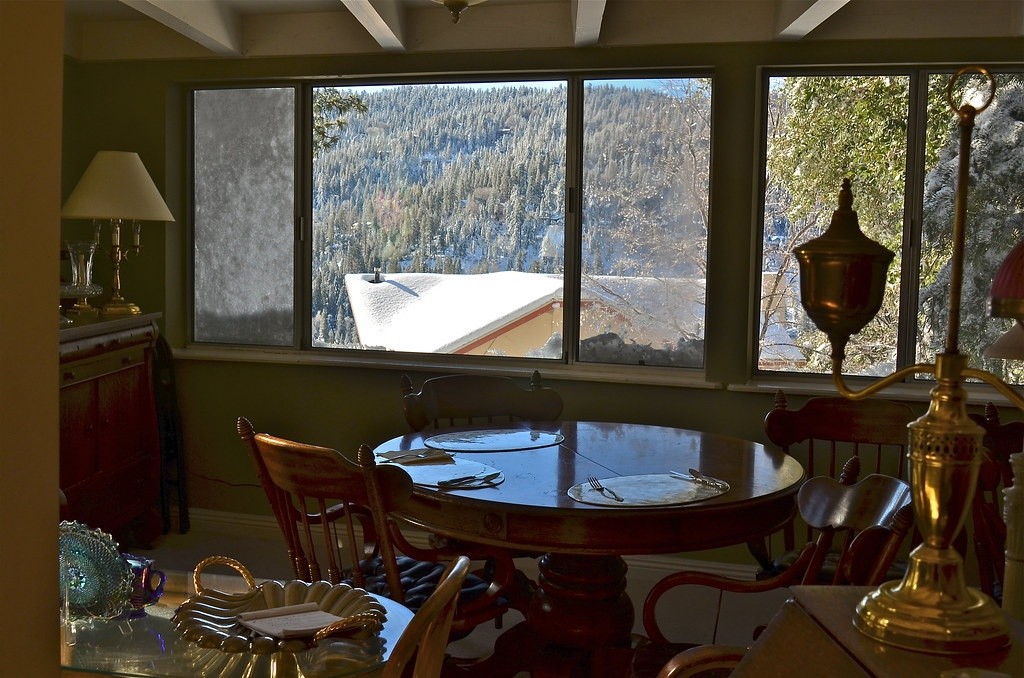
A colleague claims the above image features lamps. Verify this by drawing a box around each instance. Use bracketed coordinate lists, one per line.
[790, 67, 1024, 658]
[61, 151, 175, 313]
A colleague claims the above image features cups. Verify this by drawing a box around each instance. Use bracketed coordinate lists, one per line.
[119, 553, 166, 620]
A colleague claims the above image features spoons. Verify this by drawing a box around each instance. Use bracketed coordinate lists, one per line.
[473, 472, 500, 486]
[689, 468, 729, 489]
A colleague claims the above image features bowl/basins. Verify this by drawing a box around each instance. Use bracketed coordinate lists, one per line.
[60, 520, 135, 626]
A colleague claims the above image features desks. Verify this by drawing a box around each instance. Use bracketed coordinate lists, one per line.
[372, 419, 804, 678]
[61, 573, 417, 678]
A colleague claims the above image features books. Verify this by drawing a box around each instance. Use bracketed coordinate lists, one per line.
[237, 601, 347, 638]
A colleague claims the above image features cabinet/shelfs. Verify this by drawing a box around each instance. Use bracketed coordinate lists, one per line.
[59, 312, 168, 551]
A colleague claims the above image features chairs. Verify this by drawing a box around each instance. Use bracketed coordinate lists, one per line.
[380, 554, 471, 678]
[236, 370, 1024, 678]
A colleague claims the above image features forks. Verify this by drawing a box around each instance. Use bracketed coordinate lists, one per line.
[379, 447, 437, 464]
[587, 476, 624, 502]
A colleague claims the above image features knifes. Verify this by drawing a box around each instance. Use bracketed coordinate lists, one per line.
[438, 474, 489, 485]
[669, 470, 724, 490]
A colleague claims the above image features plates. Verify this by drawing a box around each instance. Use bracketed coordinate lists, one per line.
[172, 555, 387, 653]
